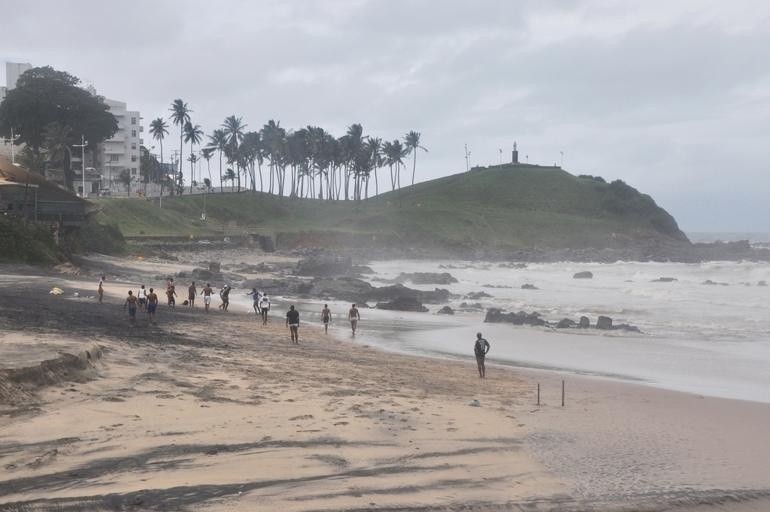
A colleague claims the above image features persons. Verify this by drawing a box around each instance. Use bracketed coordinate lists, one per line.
[260, 293, 271, 325]
[223, 287, 231, 311]
[124, 291, 139, 328]
[167, 279, 174, 288]
[188, 282, 197, 308]
[219, 285, 227, 310]
[286, 305, 300, 344]
[138, 285, 148, 314]
[321, 304, 331, 336]
[97, 276, 105, 305]
[246, 288, 262, 315]
[200, 283, 214, 314]
[348, 303, 360, 337]
[145, 288, 158, 320]
[474, 332, 490, 378]
[166, 286, 178, 308]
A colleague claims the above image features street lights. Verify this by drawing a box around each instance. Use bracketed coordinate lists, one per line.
[72, 134, 89, 198]
[0, 126, 20, 165]
[143, 144, 157, 197]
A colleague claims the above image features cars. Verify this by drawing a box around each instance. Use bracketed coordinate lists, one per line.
[97, 188, 111, 198]
[76, 191, 88, 201]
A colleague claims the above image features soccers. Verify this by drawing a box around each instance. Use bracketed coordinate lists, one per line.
[247, 311, 250, 313]
